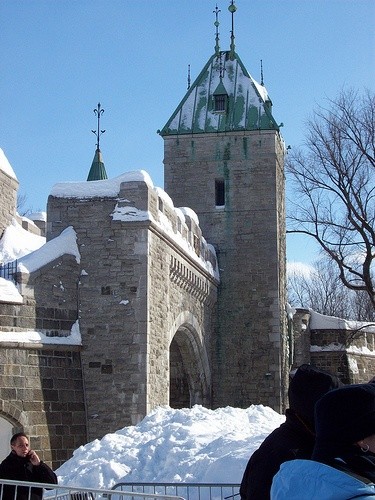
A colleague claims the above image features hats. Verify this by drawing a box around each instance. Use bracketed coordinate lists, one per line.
[314, 381, 375, 443]
[288, 364, 345, 426]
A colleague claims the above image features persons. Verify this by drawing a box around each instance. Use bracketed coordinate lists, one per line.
[236, 361, 347, 499]
[0, 432, 58, 500]
[270, 383, 375, 499]
